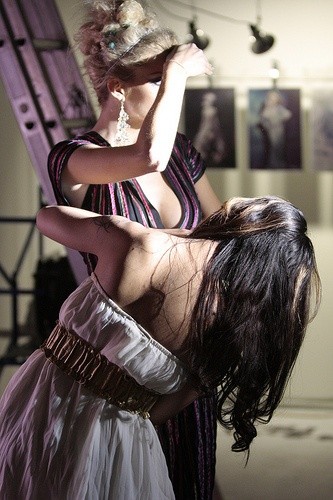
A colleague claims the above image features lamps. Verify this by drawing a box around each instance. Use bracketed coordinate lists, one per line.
[189, 22, 209, 51]
[251, 24, 274, 53]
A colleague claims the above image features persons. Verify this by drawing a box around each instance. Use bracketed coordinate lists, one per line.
[46, 1, 220, 500]
[0, 195, 326, 500]
